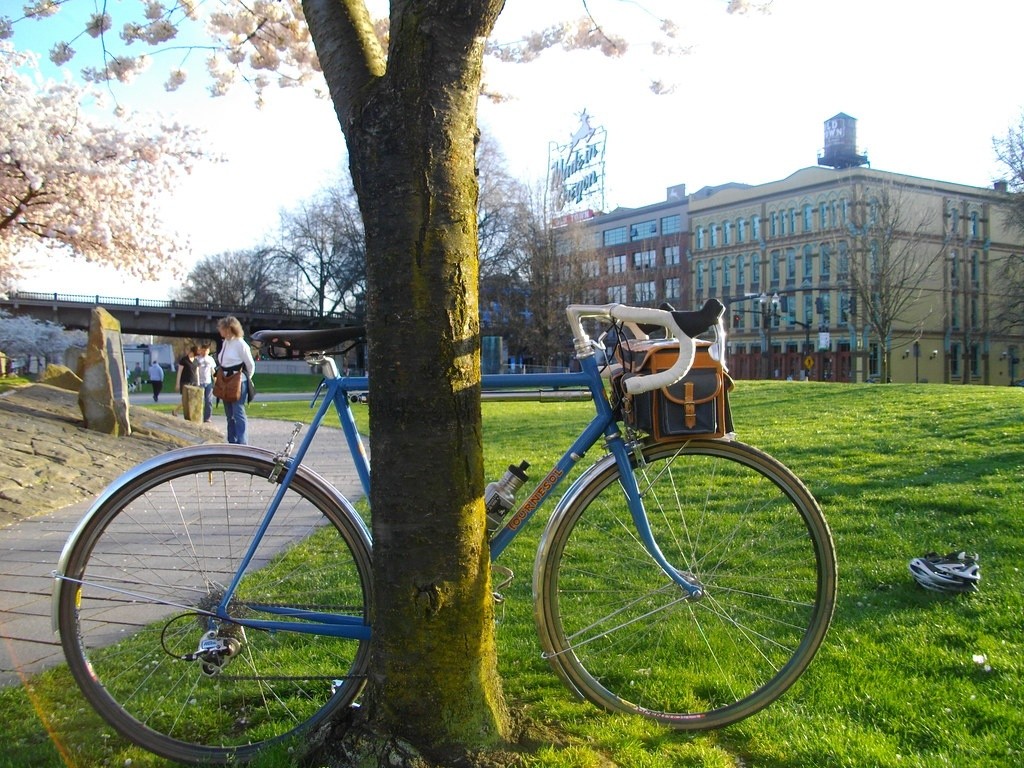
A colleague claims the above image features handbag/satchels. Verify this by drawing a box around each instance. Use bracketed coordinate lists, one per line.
[213, 368, 241, 401]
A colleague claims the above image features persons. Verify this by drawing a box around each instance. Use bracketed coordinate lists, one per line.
[176, 345, 217, 422]
[217, 316, 255, 445]
[148, 360, 164, 402]
[865, 376, 875, 383]
[787, 374, 793, 380]
[825, 370, 831, 382]
[126, 362, 143, 393]
[805, 367, 812, 381]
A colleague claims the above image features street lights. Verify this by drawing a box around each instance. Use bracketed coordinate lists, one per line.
[789, 320, 809, 382]
[914, 338, 919, 383]
[759, 289, 780, 382]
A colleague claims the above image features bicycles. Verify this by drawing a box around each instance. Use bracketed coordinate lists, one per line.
[50, 296, 838, 765]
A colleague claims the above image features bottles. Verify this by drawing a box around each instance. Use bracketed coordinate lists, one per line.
[485, 460, 531, 541]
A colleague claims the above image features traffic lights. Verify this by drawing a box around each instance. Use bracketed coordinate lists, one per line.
[733, 315, 739, 328]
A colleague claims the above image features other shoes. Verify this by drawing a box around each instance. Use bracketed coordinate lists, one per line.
[153, 395, 158, 401]
[172, 410, 178, 416]
[203, 419, 212, 422]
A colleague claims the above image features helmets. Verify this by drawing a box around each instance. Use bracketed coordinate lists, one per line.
[909, 549, 983, 591]
